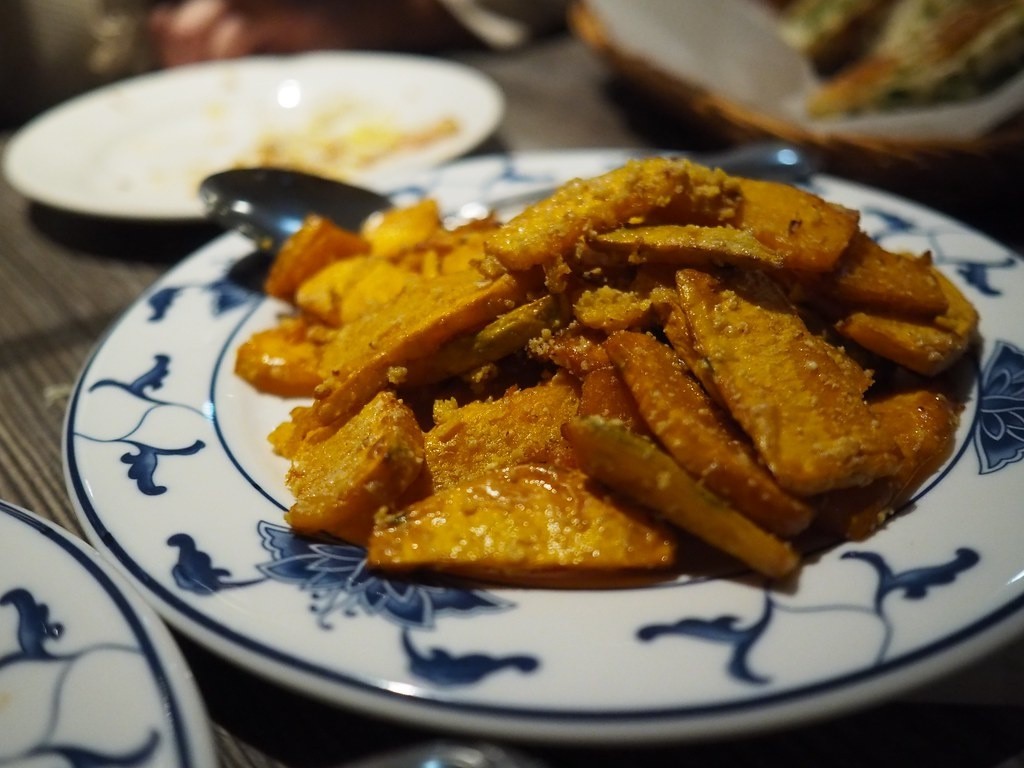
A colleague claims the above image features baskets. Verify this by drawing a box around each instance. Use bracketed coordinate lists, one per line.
[567, 1, 1024, 173]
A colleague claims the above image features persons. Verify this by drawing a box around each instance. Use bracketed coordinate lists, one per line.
[146, 0, 567, 69]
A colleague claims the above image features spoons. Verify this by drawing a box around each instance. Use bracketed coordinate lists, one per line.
[195, 142, 815, 259]
[440, 0, 529, 49]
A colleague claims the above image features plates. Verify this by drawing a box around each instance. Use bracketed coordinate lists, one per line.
[0, 498, 223, 768]
[2, 49, 507, 221]
[60, 149, 1021, 745]
